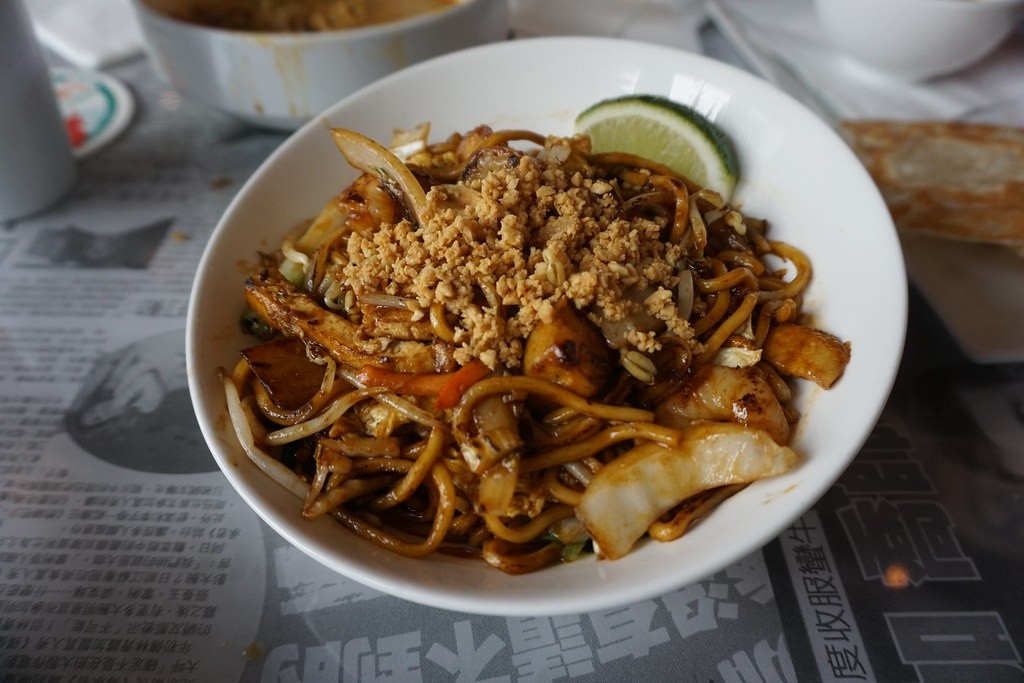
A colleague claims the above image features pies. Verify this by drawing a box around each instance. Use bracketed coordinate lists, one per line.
[852, 119, 1024, 250]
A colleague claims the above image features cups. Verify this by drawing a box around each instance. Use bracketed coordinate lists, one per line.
[0, 0, 78, 221]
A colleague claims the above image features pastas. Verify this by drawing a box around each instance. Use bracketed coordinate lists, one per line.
[216, 123, 852, 575]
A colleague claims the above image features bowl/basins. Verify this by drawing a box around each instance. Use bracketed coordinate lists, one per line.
[132, 0, 511, 130]
[814, 1, 1024, 80]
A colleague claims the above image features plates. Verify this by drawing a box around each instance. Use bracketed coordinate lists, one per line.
[185, 36, 907, 615]
[708, 0, 1024, 363]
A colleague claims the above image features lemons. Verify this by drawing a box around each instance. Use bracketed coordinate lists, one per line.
[574, 95, 737, 202]
